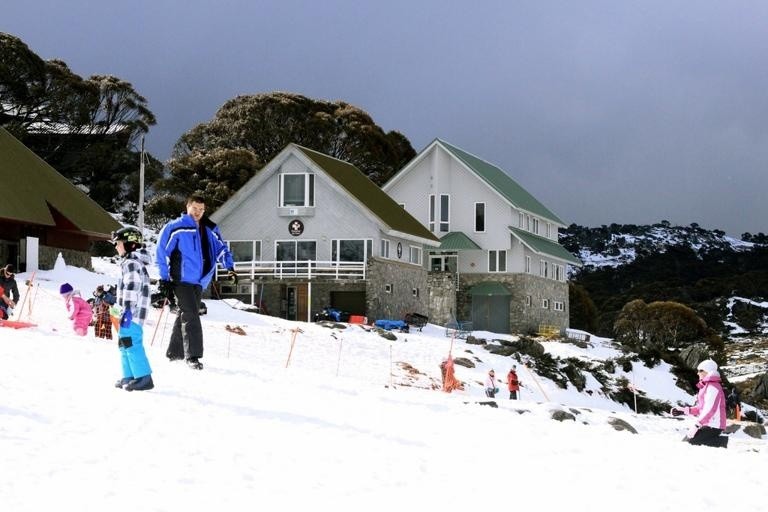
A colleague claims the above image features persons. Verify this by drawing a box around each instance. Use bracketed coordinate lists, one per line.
[728, 387, 741, 419]
[154, 196, 239, 370]
[60, 282, 93, 337]
[671, 358, 729, 448]
[0, 264, 20, 319]
[94, 284, 116, 340]
[105, 228, 153, 390]
[506, 364, 524, 400]
[484, 368, 499, 398]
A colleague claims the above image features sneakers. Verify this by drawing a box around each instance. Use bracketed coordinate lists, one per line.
[124, 376, 154, 391]
[116, 377, 134, 388]
[187, 355, 203, 370]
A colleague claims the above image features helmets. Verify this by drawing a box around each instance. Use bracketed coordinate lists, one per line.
[111, 225, 142, 248]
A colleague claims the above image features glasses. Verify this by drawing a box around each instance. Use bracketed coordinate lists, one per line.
[697, 370, 704, 372]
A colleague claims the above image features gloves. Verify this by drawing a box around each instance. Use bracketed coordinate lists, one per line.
[120, 310, 132, 328]
[226, 267, 239, 288]
[670, 406, 689, 417]
[162, 280, 176, 305]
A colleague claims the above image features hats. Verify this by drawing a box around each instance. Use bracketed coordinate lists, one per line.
[6, 264, 15, 273]
[697, 358, 718, 373]
[60, 283, 73, 294]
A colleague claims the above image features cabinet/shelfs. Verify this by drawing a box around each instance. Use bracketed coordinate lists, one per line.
[445, 319, 473, 340]
[405, 311, 429, 332]
[535, 323, 561, 341]
